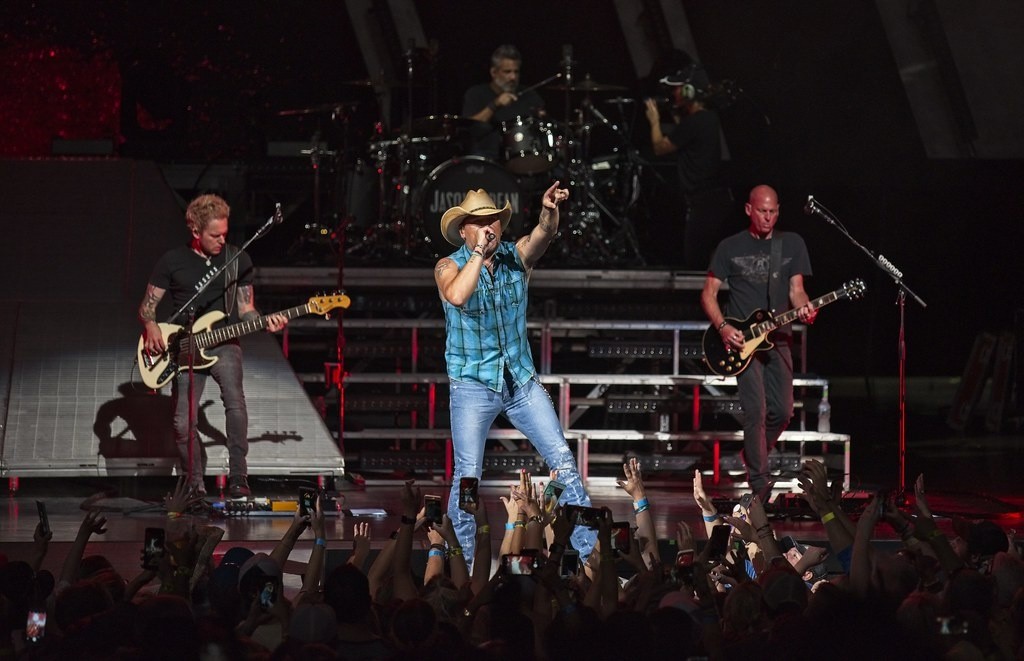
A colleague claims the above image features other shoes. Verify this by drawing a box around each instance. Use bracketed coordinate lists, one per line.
[230, 489, 248, 503]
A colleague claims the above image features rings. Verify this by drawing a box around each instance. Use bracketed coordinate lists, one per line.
[921, 490, 924, 493]
[733, 336, 737, 339]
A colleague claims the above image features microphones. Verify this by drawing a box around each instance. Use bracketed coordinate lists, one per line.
[804, 194, 814, 214]
[485, 232, 495, 241]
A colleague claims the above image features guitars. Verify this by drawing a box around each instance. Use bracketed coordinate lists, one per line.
[701, 275, 869, 378]
[136, 288, 352, 389]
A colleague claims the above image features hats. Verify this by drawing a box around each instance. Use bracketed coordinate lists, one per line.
[238, 554, 281, 587]
[952, 513, 1009, 556]
[215, 546, 256, 585]
[441, 188, 512, 248]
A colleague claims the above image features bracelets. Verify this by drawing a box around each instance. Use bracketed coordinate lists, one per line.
[449, 547, 462, 558]
[472, 251, 485, 259]
[476, 525, 490, 534]
[757, 523, 773, 538]
[314, 538, 327, 548]
[428, 550, 448, 559]
[900, 519, 915, 533]
[821, 511, 835, 525]
[719, 321, 727, 333]
[167, 512, 181, 518]
[430, 544, 446, 551]
[475, 244, 486, 250]
[902, 533, 921, 547]
[926, 527, 943, 540]
[489, 101, 498, 110]
[633, 498, 649, 512]
[514, 520, 526, 527]
[704, 510, 719, 522]
[549, 544, 565, 553]
[389, 531, 399, 539]
[401, 515, 417, 524]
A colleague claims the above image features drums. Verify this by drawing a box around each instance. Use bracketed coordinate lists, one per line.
[496, 114, 566, 177]
[414, 153, 526, 265]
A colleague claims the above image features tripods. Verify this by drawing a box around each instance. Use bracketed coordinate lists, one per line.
[123, 204, 282, 519]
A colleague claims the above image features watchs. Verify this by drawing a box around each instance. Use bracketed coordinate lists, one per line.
[529, 515, 544, 523]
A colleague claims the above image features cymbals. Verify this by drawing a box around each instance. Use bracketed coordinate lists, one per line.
[598, 97, 636, 106]
[547, 82, 631, 94]
[400, 114, 500, 144]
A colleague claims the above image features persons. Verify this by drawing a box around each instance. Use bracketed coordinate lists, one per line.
[701, 184, 819, 495]
[0, 458, 1024, 661]
[462, 44, 546, 164]
[434, 180, 599, 573]
[630, 65, 720, 249]
[138, 194, 289, 509]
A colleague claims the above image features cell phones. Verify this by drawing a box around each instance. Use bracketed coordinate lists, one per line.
[424, 494, 443, 528]
[708, 525, 732, 560]
[35, 500, 50, 535]
[566, 504, 607, 527]
[559, 550, 579, 579]
[144, 527, 165, 570]
[610, 521, 631, 559]
[543, 479, 567, 510]
[675, 548, 695, 566]
[299, 487, 317, 525]
[25, 609, 48, 645]
[458, 476, 479, 511]
[758, 481, 777, 504]
[501, 554, 540, 577]
